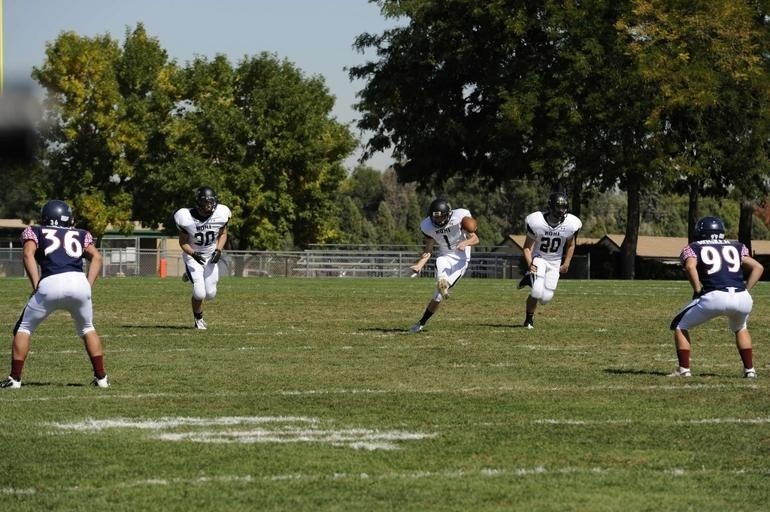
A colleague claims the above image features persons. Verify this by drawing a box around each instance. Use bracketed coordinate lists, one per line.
[171, 185, 234, 332]
[662, 214, 767, 379]
[408, 199, 480, 334]
[515, 192, 578, 329]
[0, 197, 113, 391]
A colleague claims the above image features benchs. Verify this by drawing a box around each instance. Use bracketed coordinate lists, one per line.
[293, 243, 510, 279]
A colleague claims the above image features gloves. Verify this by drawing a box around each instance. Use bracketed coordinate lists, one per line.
[191, 249, 222, 264]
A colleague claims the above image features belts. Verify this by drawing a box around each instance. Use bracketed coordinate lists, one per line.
[719, 287, 744, 293]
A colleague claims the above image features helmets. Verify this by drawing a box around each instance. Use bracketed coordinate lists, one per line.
[194, 187, 218, 218]
[547, 193, 569, 220]
[428, 199, 453, 227]
[695, 216, 726, 240]
[41, 200, 74, 225]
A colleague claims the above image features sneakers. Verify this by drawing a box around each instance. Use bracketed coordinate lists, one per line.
[745, 367, 755, 376]
[517, 271, 531, 288]
[183, 272, 190, 280]
[0, 374, 23, 389]
[194, 317, 207, 330]
[667, 365, 692, 377]
[411, 320, 424, 330]
[95, 374, 110, 389]
[523, 319, 534, 328]
[437, 277, 450, 301]
